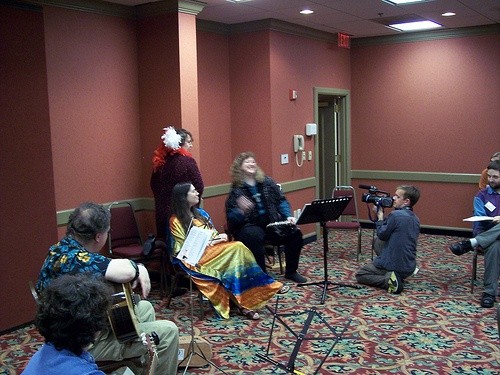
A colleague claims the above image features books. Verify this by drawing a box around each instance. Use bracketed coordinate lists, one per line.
[462, 216, 495, 222]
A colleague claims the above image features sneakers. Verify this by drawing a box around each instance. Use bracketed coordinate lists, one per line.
[449, 238, 475, 256]
[388, 271, 403, 294]
[285, 272, 307, 283]
[480, 292, 496, 308]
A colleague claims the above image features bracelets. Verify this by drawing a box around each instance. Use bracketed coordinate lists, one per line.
[137, 263, 144, 267]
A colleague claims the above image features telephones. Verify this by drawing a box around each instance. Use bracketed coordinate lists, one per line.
[294, 135, 304, 153]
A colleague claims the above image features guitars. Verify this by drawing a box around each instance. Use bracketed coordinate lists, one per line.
[104, 280, 140, 344]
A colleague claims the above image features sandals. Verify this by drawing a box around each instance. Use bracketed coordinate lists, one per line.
[236, 305, 260, 320]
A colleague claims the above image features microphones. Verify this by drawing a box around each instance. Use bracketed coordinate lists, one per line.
[359, 184, 376, 190]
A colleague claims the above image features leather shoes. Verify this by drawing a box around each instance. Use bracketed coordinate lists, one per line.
[143, 232, 157, 255]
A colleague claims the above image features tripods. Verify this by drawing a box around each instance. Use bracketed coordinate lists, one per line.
[296, 195, 362, 304]
[178, 217, 228, 375]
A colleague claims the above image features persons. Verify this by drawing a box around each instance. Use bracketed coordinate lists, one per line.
[355, 184, 421, 294]
[20, 271, 114, 375]
[150, 124, 204, 298]
[168, 182, 290, 320]
[449, 160, 500, 308]
[36, 201, 179, 375]
[226, 151, 308, 284]
[478, 152, 500, 190]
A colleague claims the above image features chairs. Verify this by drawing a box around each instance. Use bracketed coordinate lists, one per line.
[325, 185, 362, 262]
[471, 247, 483, 295]
[27, 280, 142, 375]
[167, 265, 205, 321]
[106, 201, 161, 262]
